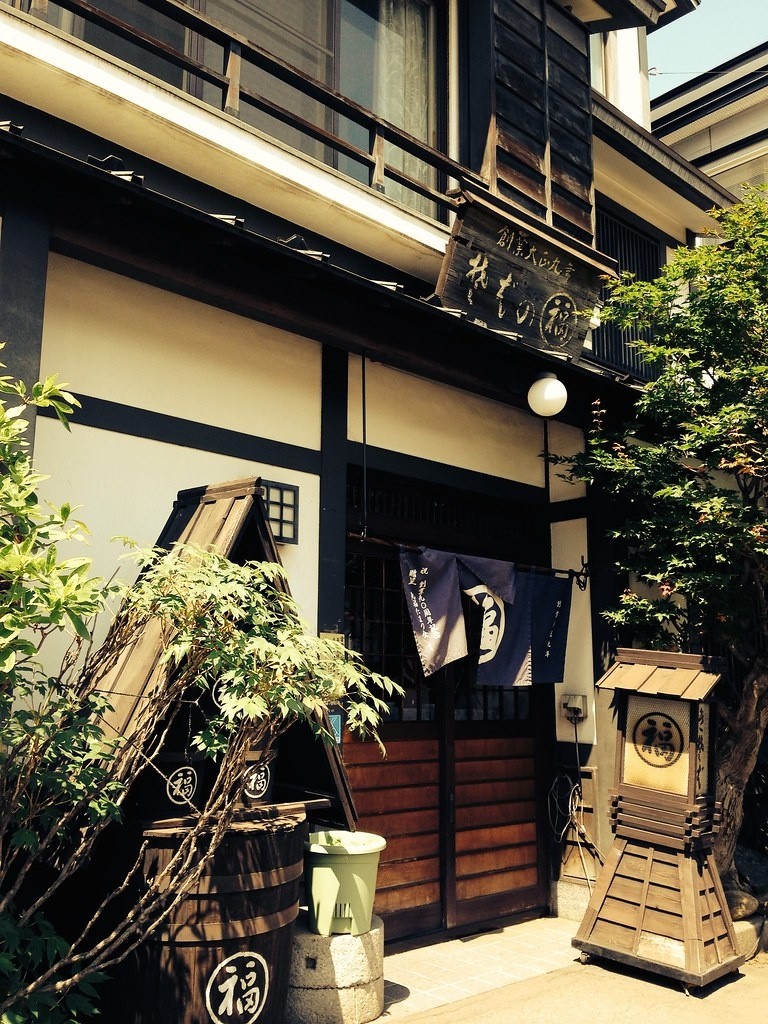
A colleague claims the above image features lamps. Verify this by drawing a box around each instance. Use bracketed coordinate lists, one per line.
[527, 362, 568, 417]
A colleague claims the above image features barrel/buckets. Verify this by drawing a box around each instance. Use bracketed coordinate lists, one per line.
[303, 830, 387, 937]
[140, 822, 308, 1024]
